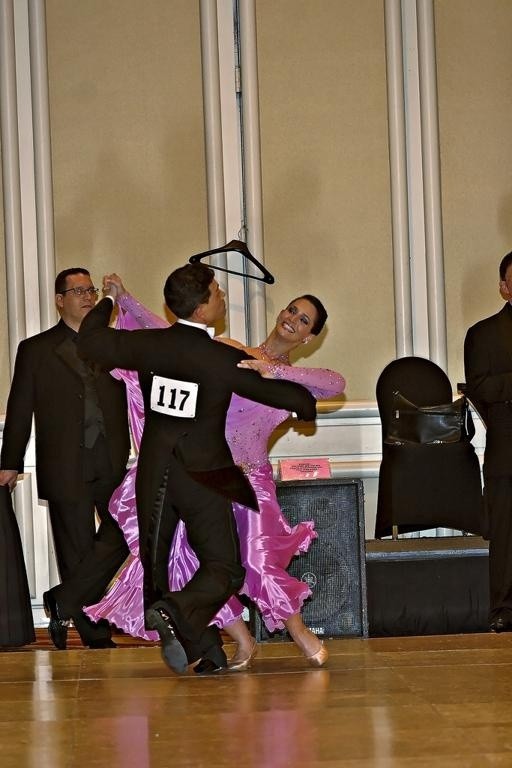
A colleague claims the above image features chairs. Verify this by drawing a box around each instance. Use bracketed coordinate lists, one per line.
[372, 355, 491, 543]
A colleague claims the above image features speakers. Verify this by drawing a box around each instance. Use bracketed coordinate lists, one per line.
[248, 476, 370, 643]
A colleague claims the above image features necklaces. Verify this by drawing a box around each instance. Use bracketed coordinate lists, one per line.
[257, 341, 289, 363]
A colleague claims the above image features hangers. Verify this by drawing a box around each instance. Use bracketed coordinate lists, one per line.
[189, 222, 280, 286]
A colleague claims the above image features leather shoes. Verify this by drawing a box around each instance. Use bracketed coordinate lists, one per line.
[193, 658, 228, 675]
[305, 629, 329, 668]
[43, 590, 68, 650]
[84, 639, 117, 648]
[227, 637, 258, 672]
[488, 617, 505, 633]
[145, 607, 191, 674]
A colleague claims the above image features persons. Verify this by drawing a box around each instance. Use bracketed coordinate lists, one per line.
[459, 250, 512, 635]
[0, 267, 131, 651]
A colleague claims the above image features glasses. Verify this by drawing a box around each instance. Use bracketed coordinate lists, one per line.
[61, 286, 99, 294]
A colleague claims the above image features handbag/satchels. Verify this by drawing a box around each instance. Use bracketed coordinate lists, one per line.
[384, 390, 465, 445]
